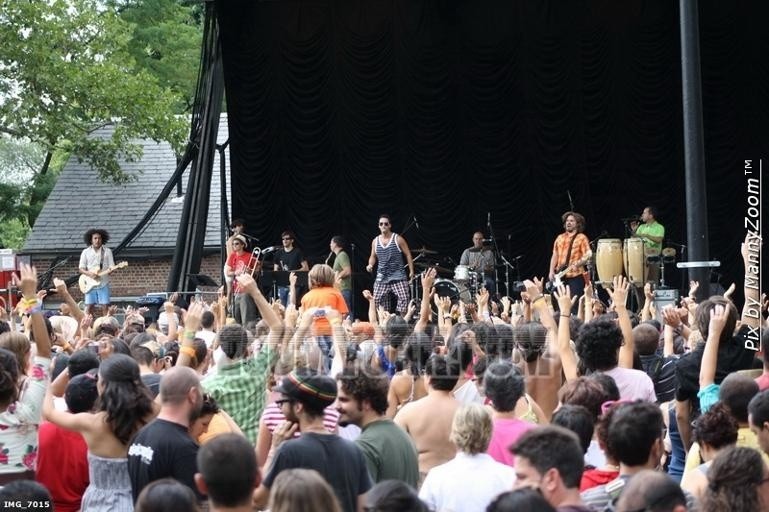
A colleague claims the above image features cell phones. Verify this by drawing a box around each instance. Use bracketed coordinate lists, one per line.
[85, 342, 105, 354]
[312, 308, 325, 318]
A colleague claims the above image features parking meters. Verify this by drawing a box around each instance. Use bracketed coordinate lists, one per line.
[227, 247, 261, 310]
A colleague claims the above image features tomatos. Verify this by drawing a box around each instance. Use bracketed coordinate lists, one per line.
[254, 270, 311, 285]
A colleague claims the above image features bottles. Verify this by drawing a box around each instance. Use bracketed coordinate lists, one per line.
[234, 234, 247, 248]
[84, 229, 109, 246]
[271, 366, 337, 408]
[49, 314, 79, 343]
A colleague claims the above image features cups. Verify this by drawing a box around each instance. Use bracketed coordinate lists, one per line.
[276, 398, 292, 409]
[231, 243, 240, 246]
[600, 399, 631, 414]
[282, 238, 292, 241]
[380, 222, 388, 226]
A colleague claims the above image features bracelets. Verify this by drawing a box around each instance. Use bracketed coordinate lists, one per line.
[673, 320, 683, 337]
[24, 297, 41, 314]
[560, 315, 569, 318]
[533, 294, 545, 306]
[179, 345, 195, 357]
[443, 313, 451, 319]
[184, 330, 195, 339]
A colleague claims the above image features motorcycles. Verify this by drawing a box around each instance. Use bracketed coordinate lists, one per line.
[471, 252, 518, 322]
[408, 256, 438, 323]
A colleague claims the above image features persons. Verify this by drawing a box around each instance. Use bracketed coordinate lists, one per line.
[549, 212, 592, 316]
[366, 215, 414, 318]
[460, 231, 497, 297]
[631, 207, 664, 312]
[0, 274, 769, 512]
[226, 236, 262, 325]
[79, 229, 128, 319]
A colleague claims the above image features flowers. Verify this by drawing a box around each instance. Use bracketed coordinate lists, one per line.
[231, 228, 241, 234]
[413, 216, 420, 231]
[487, 212, 491, 226]
[64, 255, 73, 263]
[233, 258, 237, 269]
[368, 266, 375, 275]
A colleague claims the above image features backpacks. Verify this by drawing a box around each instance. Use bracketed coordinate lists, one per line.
[453, 265, 473, 284]
[623, 237, 650, 288]
[596, 239, 623, 289]
[430, 280, 472, 318]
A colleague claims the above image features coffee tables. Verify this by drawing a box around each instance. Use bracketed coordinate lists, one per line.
[415, 263, 450, 272]
[410, 249, 438, 254]
[469, 248, 482, 252]
